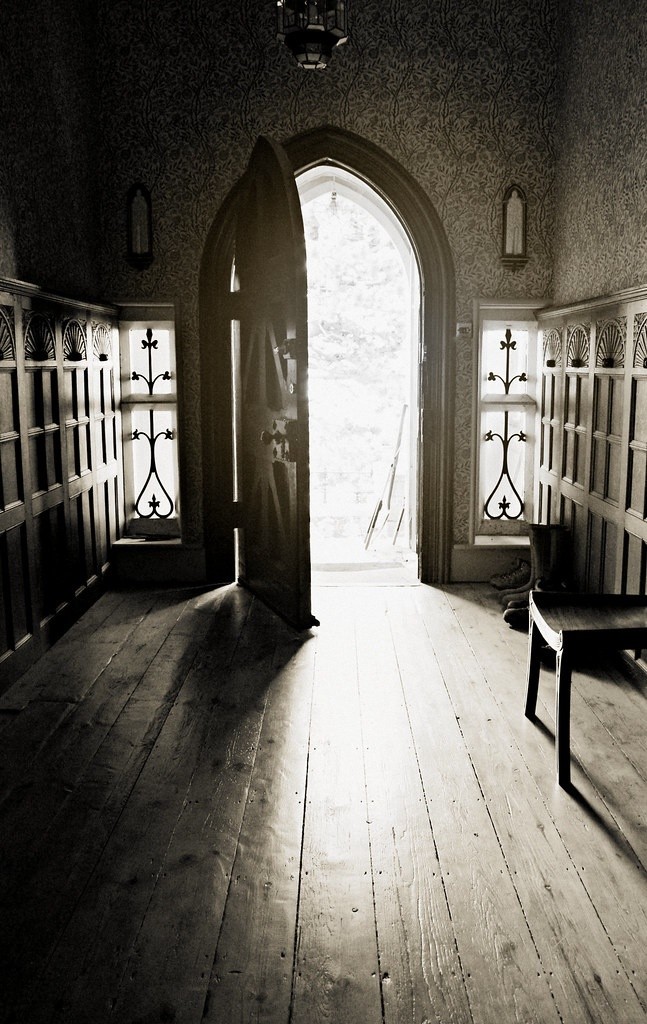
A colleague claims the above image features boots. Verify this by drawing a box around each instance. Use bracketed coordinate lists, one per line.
[497, 523, 562, 605]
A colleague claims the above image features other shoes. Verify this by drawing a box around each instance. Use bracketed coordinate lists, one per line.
[489, 559, 532, 590]
[504, 600, 529, 626]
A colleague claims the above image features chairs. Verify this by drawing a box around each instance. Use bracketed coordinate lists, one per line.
[525, 589, 647, 785]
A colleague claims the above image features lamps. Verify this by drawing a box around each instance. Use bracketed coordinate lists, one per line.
[276, 0, 348, 71]
